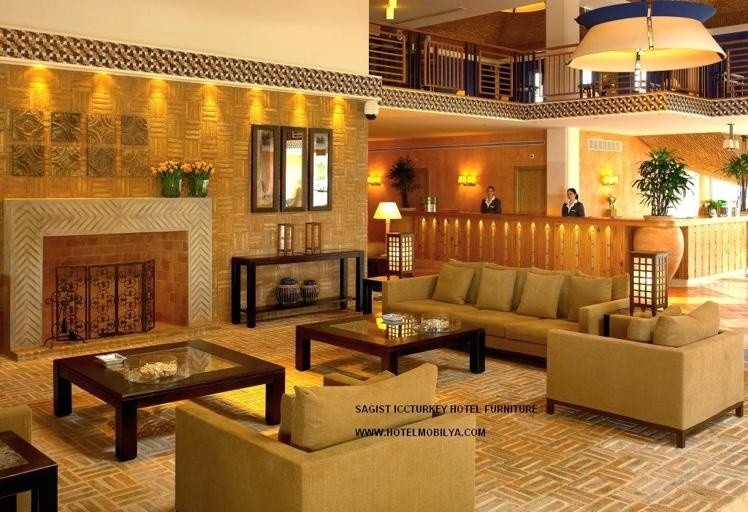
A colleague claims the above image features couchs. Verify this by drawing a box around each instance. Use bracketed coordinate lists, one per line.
[382, 257, 629, 360]
[1, 402, 37, 512]
[546, 310, 744, 447]
[175, 363, 475, 512]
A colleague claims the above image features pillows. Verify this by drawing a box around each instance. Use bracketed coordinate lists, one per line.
[273, 369, 398, 446]
[625, 302, 681, 344]
[564, 271, 612, 324]
[432, 262, 475, 305]
[516, 271, 565, 319]
[477, 267, 517, 313]
[288, 363, 439, 452]
[653, 300, 720, 349]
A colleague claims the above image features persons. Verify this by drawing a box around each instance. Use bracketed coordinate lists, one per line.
[480, 186, 501, 213]
[559, 187, 584, 216]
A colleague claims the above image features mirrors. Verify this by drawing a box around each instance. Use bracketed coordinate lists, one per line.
[308, 127, 332, 211]
[250, 125, 283, 213]
[280, 126, 308, 213]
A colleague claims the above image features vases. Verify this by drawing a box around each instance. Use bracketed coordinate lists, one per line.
[160, 179, 182, 197]
[189, 180, 209, 197]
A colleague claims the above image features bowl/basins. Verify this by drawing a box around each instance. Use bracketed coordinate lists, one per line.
[417, 313, 449, 334]
[140, 355, 177, 378]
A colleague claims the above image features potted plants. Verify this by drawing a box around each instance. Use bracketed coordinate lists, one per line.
[388, 154, 424, 212]
[632, 146, 695, 285]
[716, 152, 747, 217]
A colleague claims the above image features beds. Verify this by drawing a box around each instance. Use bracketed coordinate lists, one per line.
[53, 339, 285, 462]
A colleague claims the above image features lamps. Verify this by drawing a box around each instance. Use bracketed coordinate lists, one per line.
[305, 221, 321, 255]
[456, 173, 478, 185]
[387, 230, 417, 280]
[563, 0, 727, 74]
[720, 121, 740, 152]
[366, 174, 382, 186]
[277, 224, 295, 256]
[629, 252, 668, 316]
[599, 173, 619, 186]
[374, 201, 403, 251]
[383, 314, 415, 337]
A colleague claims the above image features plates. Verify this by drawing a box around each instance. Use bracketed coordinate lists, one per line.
[92, 351, 128, 366]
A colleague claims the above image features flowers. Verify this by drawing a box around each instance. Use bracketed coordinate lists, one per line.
[150, 160, 190, 178]
[188, 159, 215, 177]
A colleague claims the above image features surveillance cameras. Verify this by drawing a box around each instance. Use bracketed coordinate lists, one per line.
[364, 100, 379, 120]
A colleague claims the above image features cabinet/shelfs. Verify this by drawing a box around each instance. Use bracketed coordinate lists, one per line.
[362, 274, 400, 315]
[617, 306, 682, 321]
[368, 254, 413, 292]
[230, 246, 365, 328]
